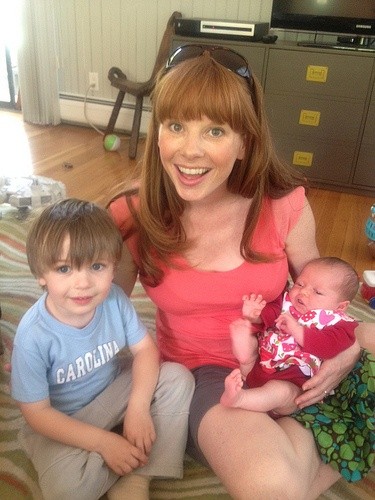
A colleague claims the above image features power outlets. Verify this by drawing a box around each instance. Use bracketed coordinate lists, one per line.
[89, 71, 100, 91]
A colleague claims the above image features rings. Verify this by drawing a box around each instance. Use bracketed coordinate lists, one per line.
[320, 400, 323, 404]
[325, 391, 328, 398]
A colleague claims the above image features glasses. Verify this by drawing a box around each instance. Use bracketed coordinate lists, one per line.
[165, 43, 254, 88]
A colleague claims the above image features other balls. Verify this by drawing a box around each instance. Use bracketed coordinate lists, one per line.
[103, 134, 120, 152]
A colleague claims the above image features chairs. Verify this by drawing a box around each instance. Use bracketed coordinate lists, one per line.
[103, 11, 181, 160]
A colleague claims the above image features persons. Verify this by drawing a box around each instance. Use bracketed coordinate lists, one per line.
[223, 258, 360, 417]
[105, 42, 375, 500]
[8, 199, 194, 500]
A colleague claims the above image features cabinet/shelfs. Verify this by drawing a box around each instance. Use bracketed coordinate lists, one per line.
[170, 38, 375, 196]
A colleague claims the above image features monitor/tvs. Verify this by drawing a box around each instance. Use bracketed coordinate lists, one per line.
[270, 0, 375, 53]
